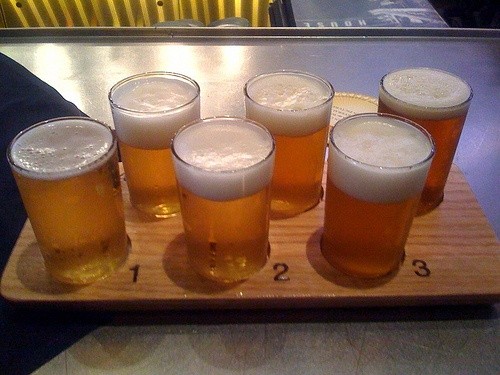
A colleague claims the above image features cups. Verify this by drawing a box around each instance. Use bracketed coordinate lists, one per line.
[376, 67, 473, 207]
[169, 115, 276, 285]
[323, 112, 437, 278]
[242, 70, 334, 216]
[107, 71, 202, 220]
[6, 116, 129, 286]
